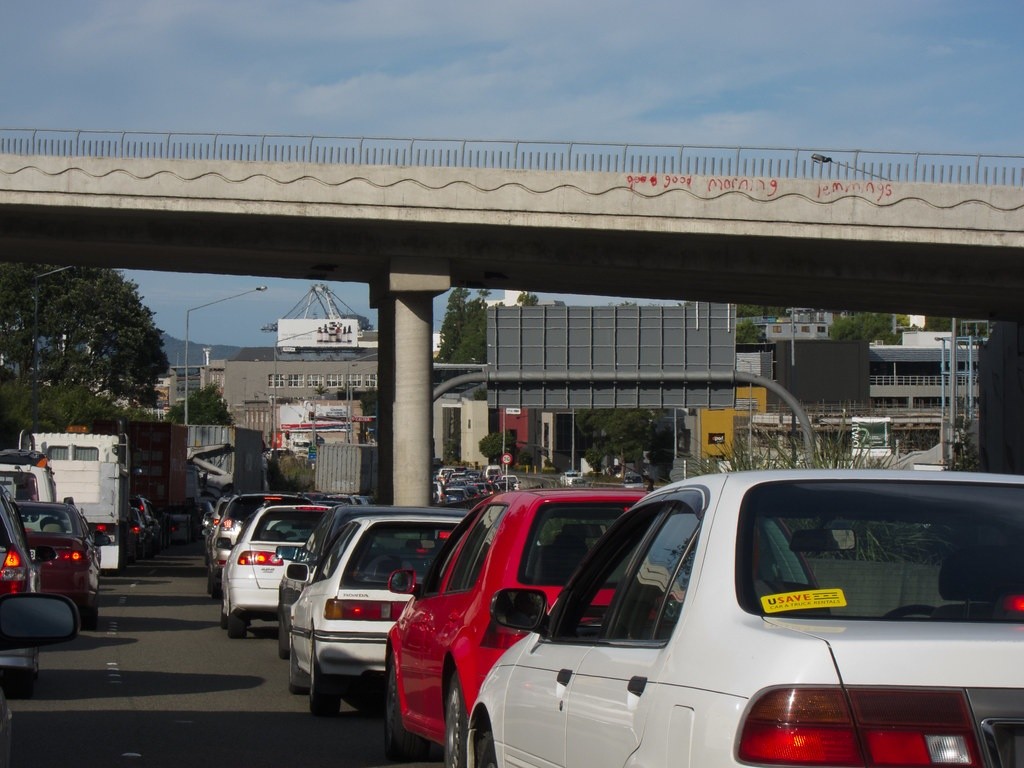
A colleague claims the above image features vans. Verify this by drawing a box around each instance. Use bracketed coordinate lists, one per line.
[483, 466, 503, 480]
[617, 470, 643, 488]
[0, 460, 59, 532]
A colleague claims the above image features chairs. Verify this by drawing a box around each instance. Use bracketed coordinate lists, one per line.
[43, 523, 63, 533]
[783, 580, 831, 613]
[931, 543, 1006, 619]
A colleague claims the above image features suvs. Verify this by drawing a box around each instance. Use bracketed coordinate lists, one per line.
[202, 493, 319, 599]
[0, 482, 56, 698]
[14, 500, 110, 630]
[215, 505, 344, 639]
[274, 505, 470, 660]
[283, 514, 475, 717]
[130, 493, 162, 552]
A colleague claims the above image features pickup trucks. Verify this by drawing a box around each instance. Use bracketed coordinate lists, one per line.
[560, 471, 584, 485]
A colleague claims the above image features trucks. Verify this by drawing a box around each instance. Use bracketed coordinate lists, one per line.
[184, 423, 264, 523]
[316, 442, 379, 496]
[27, 425, 132, 578]
[93, 417, 201, 550]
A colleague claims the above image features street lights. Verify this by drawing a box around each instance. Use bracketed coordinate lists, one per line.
[184, 286, 267, 425]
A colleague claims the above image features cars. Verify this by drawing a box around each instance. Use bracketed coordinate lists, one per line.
[432, 464, 512, 505]
[501, 474, 521, 491]
[465, 470, 1024, 768]
[380, 488, 657, 762]
[129, 505, 150, 559]
[155, 505, 177, 550]
